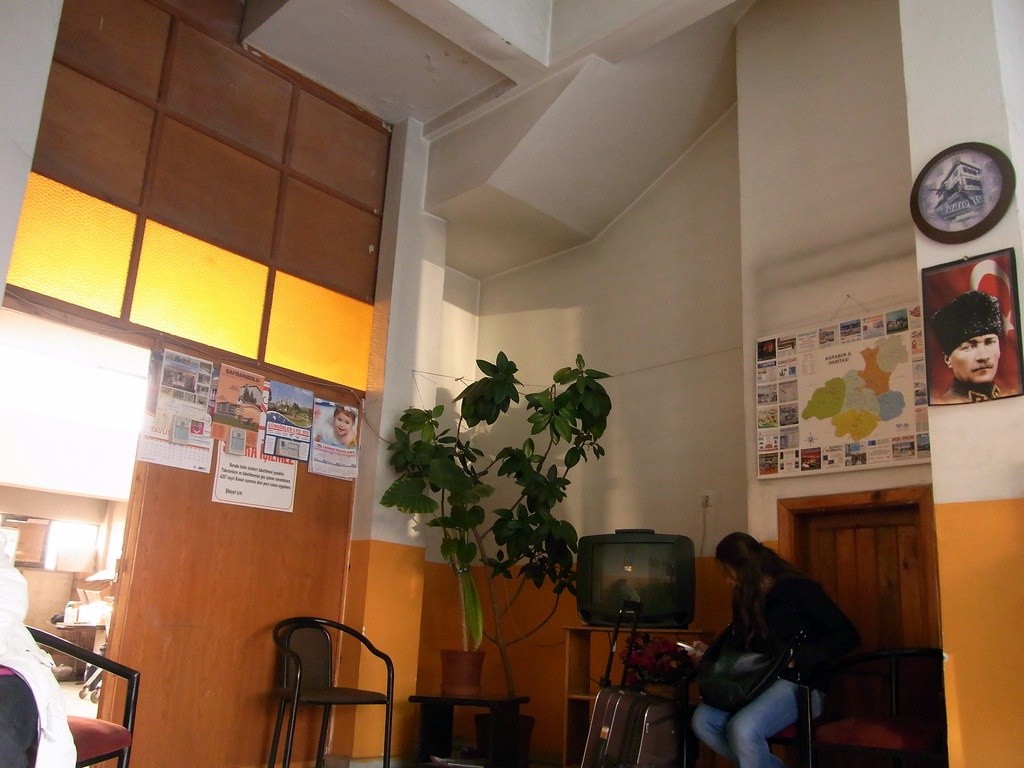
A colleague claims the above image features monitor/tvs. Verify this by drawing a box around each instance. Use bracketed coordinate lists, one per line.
[577, 534, 695, 629]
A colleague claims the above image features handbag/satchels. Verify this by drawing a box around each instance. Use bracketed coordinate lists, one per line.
[695, 622, 808, 713]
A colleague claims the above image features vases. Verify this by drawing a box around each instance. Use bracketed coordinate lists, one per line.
[646, 684, 682, 700]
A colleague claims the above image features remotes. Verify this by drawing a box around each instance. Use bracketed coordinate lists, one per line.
[614, 529, 654, 534]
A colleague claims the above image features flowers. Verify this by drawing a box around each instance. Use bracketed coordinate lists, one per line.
[619, 632, 698, 688]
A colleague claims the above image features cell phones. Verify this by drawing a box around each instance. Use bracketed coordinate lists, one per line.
[676, 641, 700, 653]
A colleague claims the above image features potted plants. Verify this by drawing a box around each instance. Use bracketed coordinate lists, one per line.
[379, 351, 613, 768]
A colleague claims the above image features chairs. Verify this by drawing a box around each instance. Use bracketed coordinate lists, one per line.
[769, 647, 947, 768]
[0, 624, 141, 768]
[268, 617, 394, 768]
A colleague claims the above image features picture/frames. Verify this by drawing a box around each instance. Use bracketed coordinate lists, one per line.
[920, 247, 1024, 406]
[909, 141, 1016, 244]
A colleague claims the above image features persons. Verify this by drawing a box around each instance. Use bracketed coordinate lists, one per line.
[690, 531, 865, 767]
[316, 407, 357, 444]
[0, 549, 78, 768]
[930, 291, 1014, 398]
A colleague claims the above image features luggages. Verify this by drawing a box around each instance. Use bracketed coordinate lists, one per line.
[574, 599, 696, 768]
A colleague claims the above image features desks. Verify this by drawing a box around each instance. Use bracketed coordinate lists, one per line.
[407, 695, 531, 768]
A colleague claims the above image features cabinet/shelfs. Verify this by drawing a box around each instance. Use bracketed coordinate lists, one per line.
[563, 626, 720, 768]
